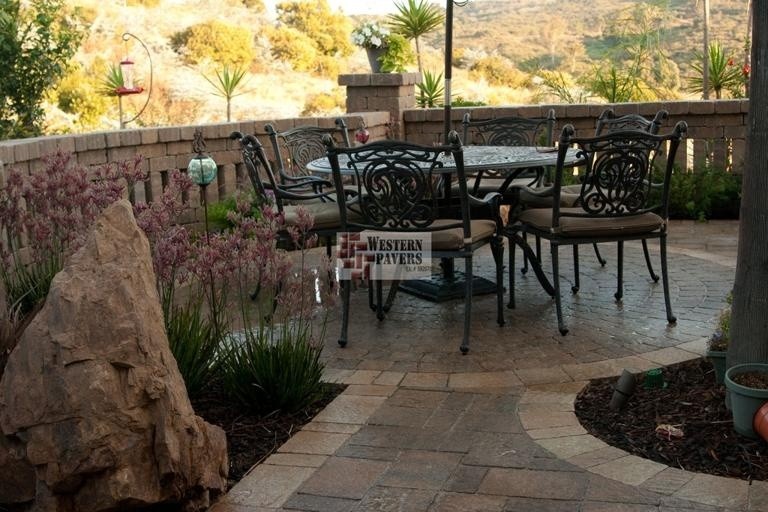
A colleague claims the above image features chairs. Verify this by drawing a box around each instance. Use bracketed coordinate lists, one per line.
[229, 130, 377, 322]
[516, 110, 669, 282]
[261, 118, 362, 204]
[503, 121, 689, 338]
[450, 109, 557, 274]
[317, 131, 508, 356]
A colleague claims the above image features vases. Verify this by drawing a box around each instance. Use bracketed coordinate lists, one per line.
[752, 399, 768, 443]
[706, 351, 726, 382]
[365, 46, 390, 74]
[724, 362, 768, 438]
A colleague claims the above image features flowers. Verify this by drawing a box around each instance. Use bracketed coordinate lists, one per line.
[706, 290, 733, 350]
[350, 19, 419, 73]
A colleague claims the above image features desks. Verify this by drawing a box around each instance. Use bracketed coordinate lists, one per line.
[305, 142, 591, 303]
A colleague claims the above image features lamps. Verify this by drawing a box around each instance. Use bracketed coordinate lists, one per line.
[113, 31, 154, 129]
